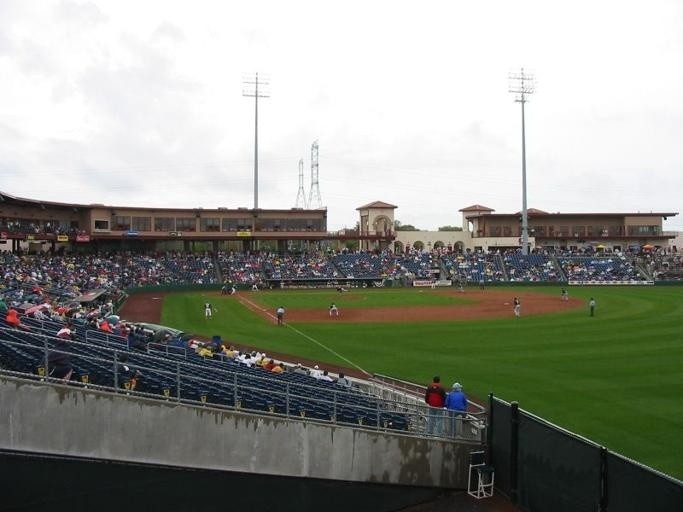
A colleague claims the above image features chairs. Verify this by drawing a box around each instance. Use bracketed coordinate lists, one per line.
[1, 254, 122, 312]
[468, 450, 496, 500]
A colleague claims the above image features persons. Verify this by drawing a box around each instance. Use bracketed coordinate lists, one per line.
[426, 375, 446, 435]
[1, 284, 363, 393]
[513, 296, 521, 318]
[1, 215, 683, 289]
[446, 383, 468, 437]
[561, 287, 569, 300]
[589, 297, 595, 316]
